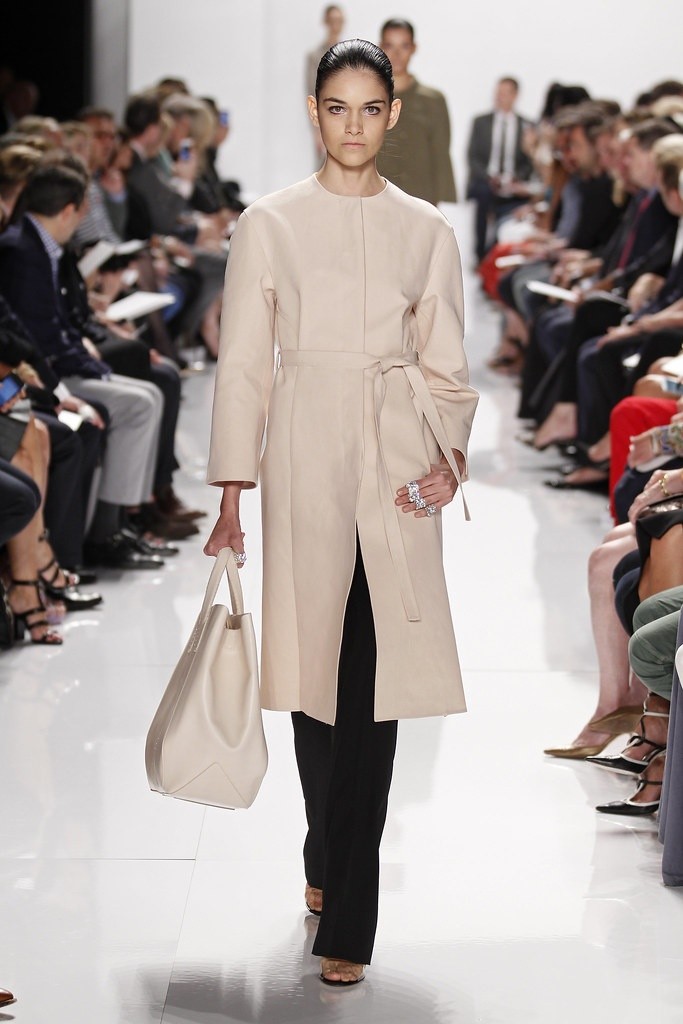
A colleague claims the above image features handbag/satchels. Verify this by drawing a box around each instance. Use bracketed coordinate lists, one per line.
[145, 546, 268, 811]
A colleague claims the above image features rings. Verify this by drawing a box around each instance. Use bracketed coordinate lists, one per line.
[662, 489, 670, 496]
[416, 498, 426, 509]
[659, 474, 668, 481]
[404, 481, 419, 494]
[425, 504, 437, 517]
[233, 552, 247, 563]
[407, 492, 420, 502]
[659, 481, 665, 489]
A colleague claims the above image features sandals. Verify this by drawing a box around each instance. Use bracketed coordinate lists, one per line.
[319, 959, 366, 986]
[305, 883, 323, 914]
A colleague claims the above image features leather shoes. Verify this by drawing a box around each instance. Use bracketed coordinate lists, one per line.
[81, 533, 164, 569]
[123, 530, 179, 557]
[45, 585, 103, 611]
[1, 990, 17, 1007]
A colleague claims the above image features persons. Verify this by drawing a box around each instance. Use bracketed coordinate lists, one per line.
[460, 76, 539, 274]
[0, 77, 249, 647]
[304, 5, 345, 173]
[204, 40, 482, 985]
[372, 16, 459, 209]
[472, 81, 683, 886]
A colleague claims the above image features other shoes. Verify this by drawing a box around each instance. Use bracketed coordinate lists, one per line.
[572, 448, 611, 467]
[542, 475, 609, 496]
[147, 499, 207, 540]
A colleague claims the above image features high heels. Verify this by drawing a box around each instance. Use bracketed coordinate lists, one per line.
[515, 432, 577, 455]
[7, 579, 64, 646]
[596, 750, 667, 818]
[544, 704, 645, 759]
[586, 691, 671, 776]
[37, 528, 80, 590]
